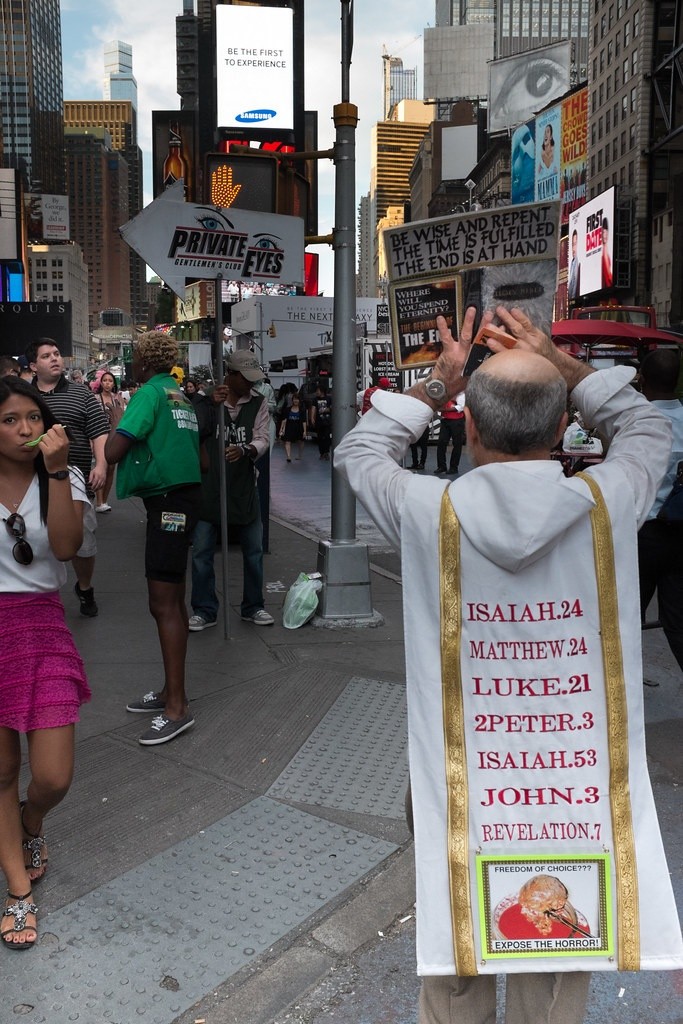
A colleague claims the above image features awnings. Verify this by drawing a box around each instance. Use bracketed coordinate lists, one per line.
[297, 348, 333, 365]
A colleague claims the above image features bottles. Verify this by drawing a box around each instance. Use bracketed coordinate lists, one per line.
[164, 140, 185, 197]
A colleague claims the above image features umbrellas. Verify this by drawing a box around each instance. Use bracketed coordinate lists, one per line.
[550, 319, 683, 363]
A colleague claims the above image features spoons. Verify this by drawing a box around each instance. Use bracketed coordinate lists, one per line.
[25, 425, 67, 447]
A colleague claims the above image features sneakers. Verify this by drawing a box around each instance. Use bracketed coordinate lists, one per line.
[74, 580, 99, 617]
[187, 615, 218, 631]
[126, 691, 167, 712]
[241, 610, 275, 625]
[139, 712, 195, 744]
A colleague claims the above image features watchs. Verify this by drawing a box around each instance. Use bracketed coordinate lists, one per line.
[48, 470, 69, 480]
[241, 444, 252, 457]
[424, 375, 449, 408]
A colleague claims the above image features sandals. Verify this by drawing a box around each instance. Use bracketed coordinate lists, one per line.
[0, 886, 38, 949]
[17, 799, 49, 886]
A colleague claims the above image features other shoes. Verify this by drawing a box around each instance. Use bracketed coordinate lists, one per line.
[287, 457, 291, 463]
[319, 452, 331, 461]
[405, 463, 425, 470]
[95, 502, 112, 512]
[433, 466, 448, 473]
[445, 466, 459, 474]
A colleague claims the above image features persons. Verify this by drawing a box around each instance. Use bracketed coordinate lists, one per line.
[602, 217, 612, 288]
[0, 376, 92, 949]
[569, 230, 580, 300]
[333, 306, 683, 1024]
[537, 124, 558, 174]
[486, 42, 571, 131]
[409, 391, 465, 475]
[638, 349, 683, 669]
[0, 337, 330, 632]
[104, 331, 202, 747]
[357, 378, 402, 425]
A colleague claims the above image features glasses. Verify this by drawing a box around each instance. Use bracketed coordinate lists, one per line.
[3, 513, 34, 565]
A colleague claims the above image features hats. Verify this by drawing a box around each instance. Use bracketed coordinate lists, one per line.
[378, 378, 392, 389]
[230, 348, 267, 382]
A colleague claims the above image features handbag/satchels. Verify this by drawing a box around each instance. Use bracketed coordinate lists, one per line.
[279, 418, 286, 436]
[281, 570, 324, 629]
